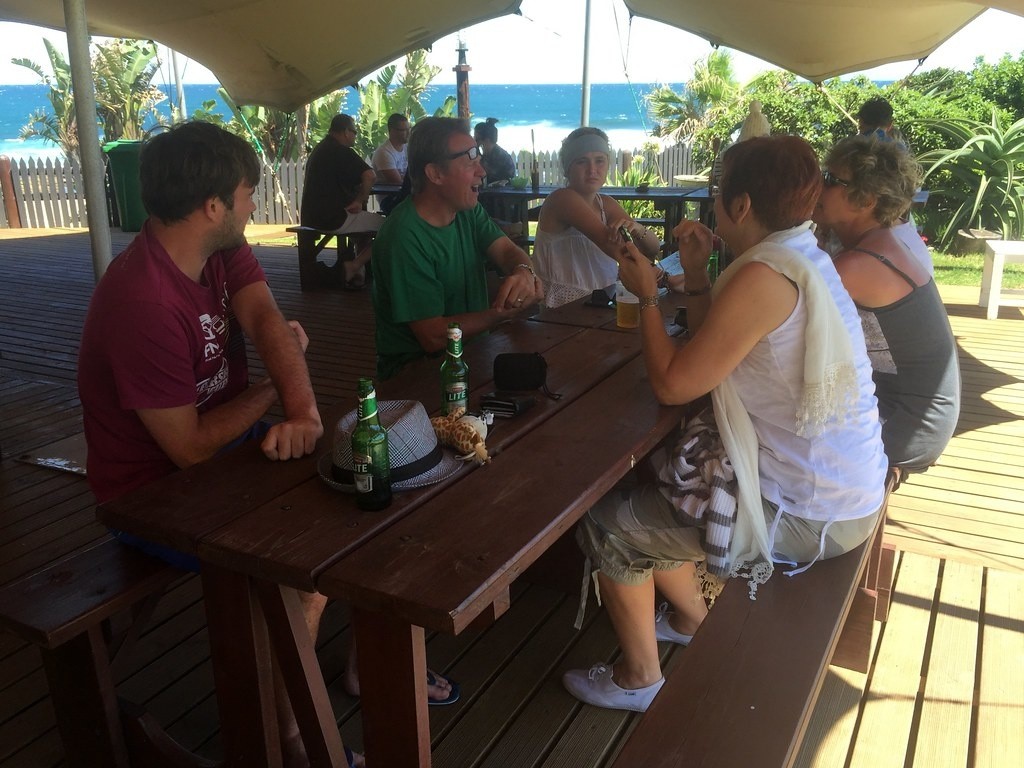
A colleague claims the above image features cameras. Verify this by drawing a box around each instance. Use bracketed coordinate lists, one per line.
[619, 224, 634, 244]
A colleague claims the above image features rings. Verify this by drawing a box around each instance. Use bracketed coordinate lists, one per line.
[517, 299, 524, 302]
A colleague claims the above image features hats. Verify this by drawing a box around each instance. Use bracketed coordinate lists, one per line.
[736, 100, 770, 143]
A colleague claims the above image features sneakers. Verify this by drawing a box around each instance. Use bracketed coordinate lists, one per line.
[562, 662, 666, 712]
[654, 601, 695, 646]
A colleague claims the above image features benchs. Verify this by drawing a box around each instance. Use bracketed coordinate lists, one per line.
[286, 224, 534, 295]
[613, 460, 898, 768]
[0, 534, 193, 768]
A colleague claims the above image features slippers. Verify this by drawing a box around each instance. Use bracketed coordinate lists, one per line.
[341, 746, 358, 768]
[342, 664, 459, 705]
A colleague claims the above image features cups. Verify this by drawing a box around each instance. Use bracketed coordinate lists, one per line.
[532, 172, 540, 189]
[615, 281, 640, 328]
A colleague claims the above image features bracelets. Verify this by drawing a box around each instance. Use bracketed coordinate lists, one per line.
[638, 296, 660, 312]
[513, 264, 535, 277]
[683, 281, 712, 296]
[632, 226, 648, 240]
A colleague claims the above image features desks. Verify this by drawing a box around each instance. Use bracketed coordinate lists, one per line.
[372, 185, 928, 262]
[95, 255, 710, 768]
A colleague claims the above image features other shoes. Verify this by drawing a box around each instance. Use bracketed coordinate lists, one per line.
[332, 258, 358, 293]
[354, 264, 368, 288]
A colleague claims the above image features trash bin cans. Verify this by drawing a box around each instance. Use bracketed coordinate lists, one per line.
[100, 139, 148, 233]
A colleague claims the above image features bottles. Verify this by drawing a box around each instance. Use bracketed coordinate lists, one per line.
[706, 234, 718, 287]
[440, 322, 471, 417]
[352, 377, 392, 509]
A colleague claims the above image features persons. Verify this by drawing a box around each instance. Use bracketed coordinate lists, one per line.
[372, 113, 411, 216]
[564, 136, 892, 716]
[857, 98, 894, 138]
[533, 127, 661, 312]
[77, 121, 461, 768]
[475, 118, 515, 213]
[812, 137, 960, 472]
[371, 117, 545, 385]
[301, 114, 385, 289]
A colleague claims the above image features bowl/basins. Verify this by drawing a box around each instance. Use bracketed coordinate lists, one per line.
[510, 178, 527, 190]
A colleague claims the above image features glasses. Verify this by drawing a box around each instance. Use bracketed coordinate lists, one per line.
[343, 127, 357, 135]
[823, 171, 848, 189]
[435, 144, 485, 164]
[392, 127, 411, 132]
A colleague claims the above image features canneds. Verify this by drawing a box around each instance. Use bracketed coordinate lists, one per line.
[706, 253, 718, 282]
[480, 176, 488, 190]
